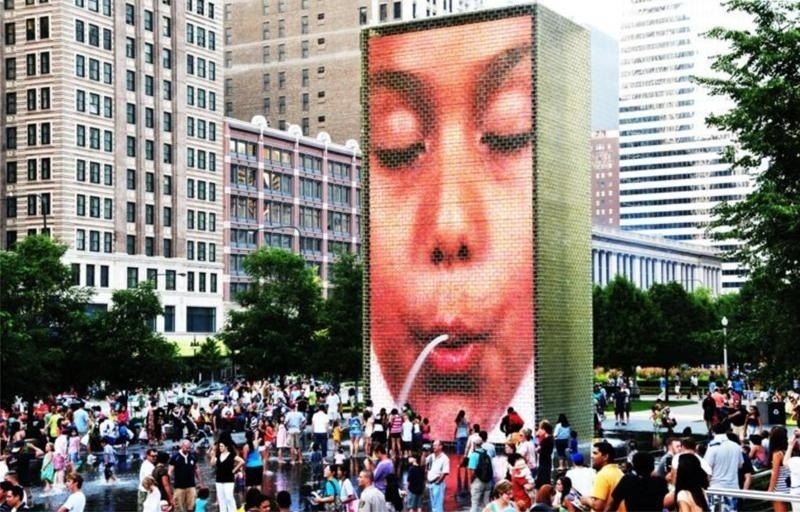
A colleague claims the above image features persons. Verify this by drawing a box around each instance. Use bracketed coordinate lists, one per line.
[366, 16, 533, 439]
[1, 366, 800, 512]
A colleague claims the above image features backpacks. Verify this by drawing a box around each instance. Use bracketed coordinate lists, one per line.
[478, 454, 493, 483]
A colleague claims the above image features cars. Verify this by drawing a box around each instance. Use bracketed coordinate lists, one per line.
[57, 395, 99, 409]
[188, 381, 223, 399]
[128, 394, 148, 411]
[173, 381, 197, 397]
[598, 437, 629, 465]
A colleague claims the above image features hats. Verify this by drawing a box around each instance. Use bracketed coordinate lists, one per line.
[567, 452, 584, 462]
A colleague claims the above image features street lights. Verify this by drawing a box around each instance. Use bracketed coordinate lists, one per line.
[148, 269, 185, 294]
[721, 315, 730, 379]
[245, 224, 303, 257]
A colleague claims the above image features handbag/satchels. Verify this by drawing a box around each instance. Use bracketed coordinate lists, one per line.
[324, 497, 342, 511]
